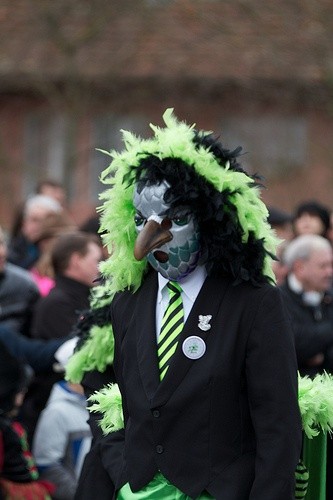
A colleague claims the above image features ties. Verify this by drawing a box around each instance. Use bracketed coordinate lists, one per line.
[158, 282, 184, 382]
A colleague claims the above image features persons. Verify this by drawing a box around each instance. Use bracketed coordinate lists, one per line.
[277, 235, 333, 380]
[22, 232, 103, 452]
[64, 108, 303, 500]
[267, 201, 333, 288]
[0, 180, 110, 500]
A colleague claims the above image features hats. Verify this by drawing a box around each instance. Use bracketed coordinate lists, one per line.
[53, 336, 80, 372]
